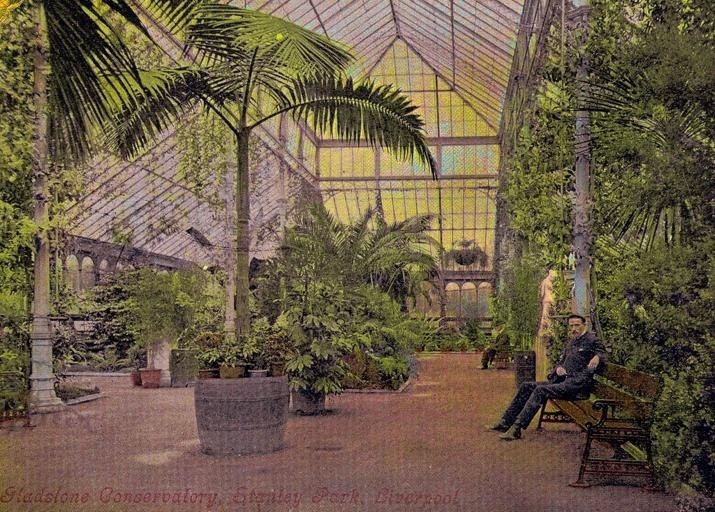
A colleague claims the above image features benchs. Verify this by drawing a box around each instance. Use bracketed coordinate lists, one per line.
[535, 360, 668, 492]
[496, 343, 517, 369]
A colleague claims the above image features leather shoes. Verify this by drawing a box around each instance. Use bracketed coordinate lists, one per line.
[489, 419, 510, 433]
[498, 423, 522, 440]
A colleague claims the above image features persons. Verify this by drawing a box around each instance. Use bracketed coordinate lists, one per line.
[484, 314, 606, 440]
[476, 319, 510, 370]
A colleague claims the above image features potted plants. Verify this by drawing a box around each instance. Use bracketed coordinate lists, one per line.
[95, 1, 495, 459]
